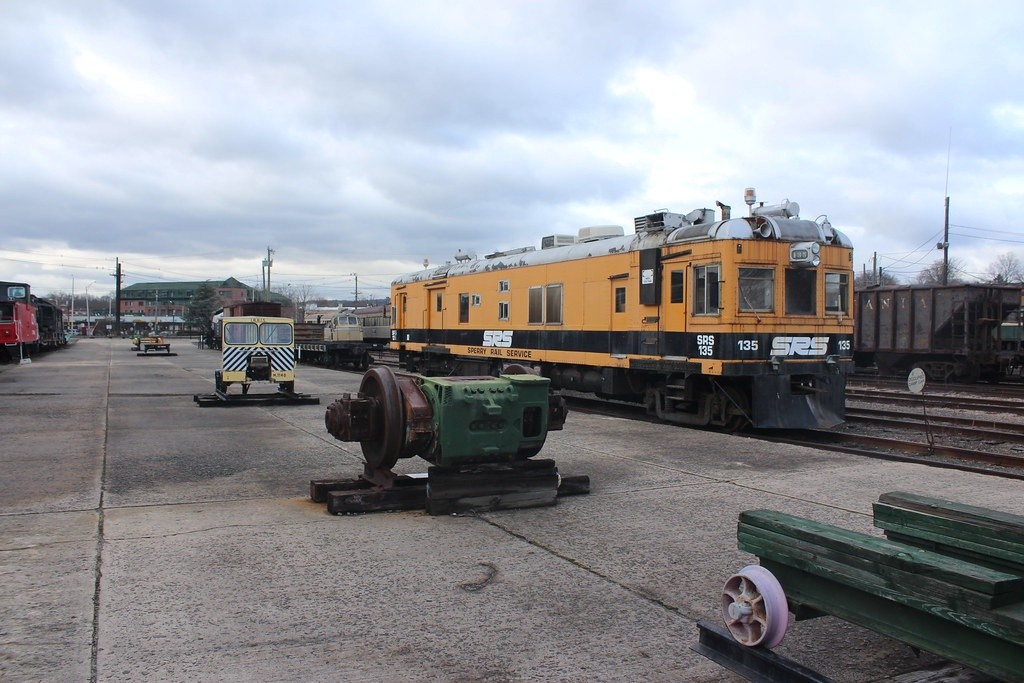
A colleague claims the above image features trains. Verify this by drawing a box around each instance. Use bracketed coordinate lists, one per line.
[304, 305, 391, 346]
[389, 189, 854, 430]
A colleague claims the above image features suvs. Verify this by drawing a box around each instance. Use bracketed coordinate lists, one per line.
[65, 329, 79, 336]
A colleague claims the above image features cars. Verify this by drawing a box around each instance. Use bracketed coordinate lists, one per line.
[160, 331, 172, 336]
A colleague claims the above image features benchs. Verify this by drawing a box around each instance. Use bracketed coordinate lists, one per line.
[144, 344, 170, 353]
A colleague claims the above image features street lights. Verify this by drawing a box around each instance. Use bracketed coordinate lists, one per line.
[86, 281, 96, 329]
